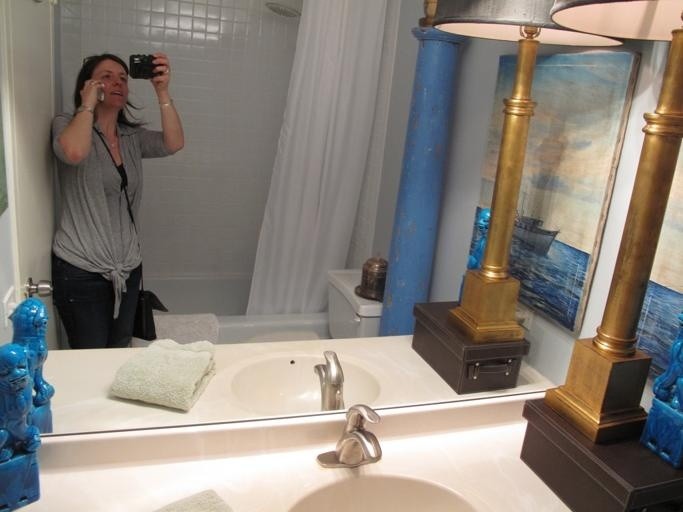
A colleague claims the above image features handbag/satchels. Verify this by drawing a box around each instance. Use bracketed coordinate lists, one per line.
[134, 286, 169, 342]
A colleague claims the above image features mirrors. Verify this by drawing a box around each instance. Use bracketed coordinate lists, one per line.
[2, 0, 644, 440]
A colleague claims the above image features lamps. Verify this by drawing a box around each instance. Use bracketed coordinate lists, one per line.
[413, 0, 623, 344]
[520, 0, 681, 446]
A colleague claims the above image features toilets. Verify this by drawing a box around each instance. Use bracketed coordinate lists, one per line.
[238, 271, 385, 342]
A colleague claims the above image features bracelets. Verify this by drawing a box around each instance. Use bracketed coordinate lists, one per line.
[76, 104, 94, 114]
[157, 98, 173, 107]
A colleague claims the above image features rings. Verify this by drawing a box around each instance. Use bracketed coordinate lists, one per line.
[163, 69, 169, 74]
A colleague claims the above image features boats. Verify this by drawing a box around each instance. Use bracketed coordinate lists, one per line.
[514, 191, 560, 256]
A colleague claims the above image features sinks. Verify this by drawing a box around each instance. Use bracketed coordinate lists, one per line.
[226, 352, 383, 418]
[282, 471, 487, 512]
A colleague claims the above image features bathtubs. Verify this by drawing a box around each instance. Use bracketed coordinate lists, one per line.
[135, 270, 331, 343]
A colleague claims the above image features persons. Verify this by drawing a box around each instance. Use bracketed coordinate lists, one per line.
[47, 50, 186, 350]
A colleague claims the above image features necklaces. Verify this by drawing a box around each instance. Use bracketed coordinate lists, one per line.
[95, 124, 116, 150]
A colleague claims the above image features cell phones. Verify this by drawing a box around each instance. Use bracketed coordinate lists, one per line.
[94, 84, 105, 101]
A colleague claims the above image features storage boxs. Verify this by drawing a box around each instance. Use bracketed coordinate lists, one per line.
[517, 400, 682, 512]
[410, 295, 534, 396]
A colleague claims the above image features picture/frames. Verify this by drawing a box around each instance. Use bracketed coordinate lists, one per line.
[457, 53, 644, 339]
[636, 141, 683, 371]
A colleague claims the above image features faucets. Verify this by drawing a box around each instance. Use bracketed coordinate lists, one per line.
[315, 403, 385, 467]
[311, 351, 347, 414]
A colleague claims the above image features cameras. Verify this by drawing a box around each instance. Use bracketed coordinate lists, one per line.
[128, 53, 162, 80]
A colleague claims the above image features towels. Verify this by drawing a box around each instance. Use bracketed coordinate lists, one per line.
[106, 337, 222, 412]
[155, 490, 229, 512]
